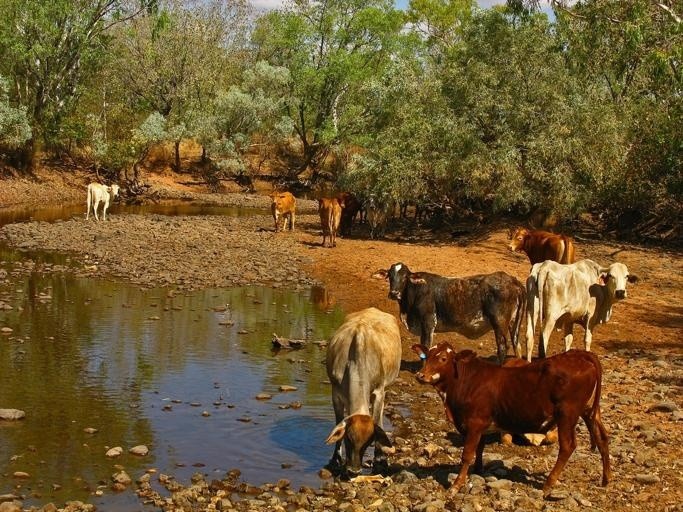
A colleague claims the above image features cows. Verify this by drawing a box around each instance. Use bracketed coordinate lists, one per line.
[454, 348, 597, 453]
[524, 258, 638, 364]
[370, 262, 526, 368]
[337, 190, 359, 238]
[85, 182, 121, 221]
[411, 340, 611, 503]
[324, 307, 403, 480]
[268, 190, 296, 233]
[507, 225, 576, 266]
[317, 196, 342, 248]
